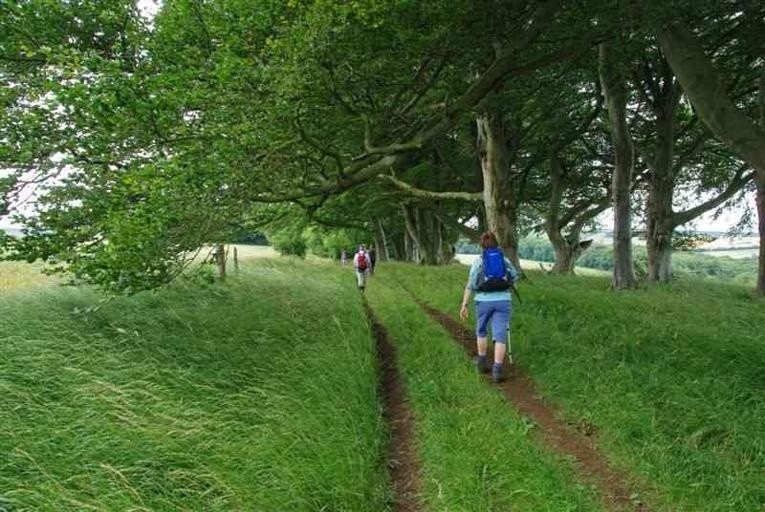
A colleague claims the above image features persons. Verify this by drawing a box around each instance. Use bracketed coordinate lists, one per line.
[341, 243, 376, 293]
[459, 231, 520, 384]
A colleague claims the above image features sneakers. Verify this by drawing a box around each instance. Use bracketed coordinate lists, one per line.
[473, 355, 487, 364]
[492, 365, 502, 379]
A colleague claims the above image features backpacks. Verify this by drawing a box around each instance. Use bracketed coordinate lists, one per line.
[478, 247, 509, 292]
[358, 253, 366, 268]
